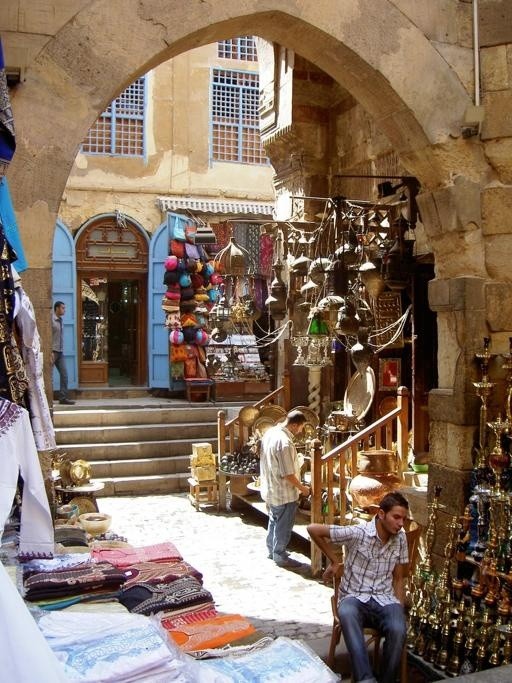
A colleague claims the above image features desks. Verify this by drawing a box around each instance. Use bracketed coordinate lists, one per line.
[56, 482, 105, 505]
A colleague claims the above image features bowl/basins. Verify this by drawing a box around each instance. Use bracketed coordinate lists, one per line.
[79, 512, 113, 534]
[55, 504, 78, 520]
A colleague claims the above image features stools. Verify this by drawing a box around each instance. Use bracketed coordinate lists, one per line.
[187, 477, 219, 513]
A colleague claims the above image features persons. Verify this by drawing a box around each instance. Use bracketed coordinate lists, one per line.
[260, 410, 310, 568]
[52, 301, 76, 405]
[307, 492, 411, 683]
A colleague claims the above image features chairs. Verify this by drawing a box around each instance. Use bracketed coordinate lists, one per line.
[323, 566, 408, 683]
[181, 379, 212, 401]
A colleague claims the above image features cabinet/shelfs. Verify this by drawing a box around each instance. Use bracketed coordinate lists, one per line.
[211, 237, 270, 404]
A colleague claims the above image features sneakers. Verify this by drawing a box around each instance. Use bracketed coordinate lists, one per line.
[276, 558, 302, 567]
[59, 399, 75, 405]
